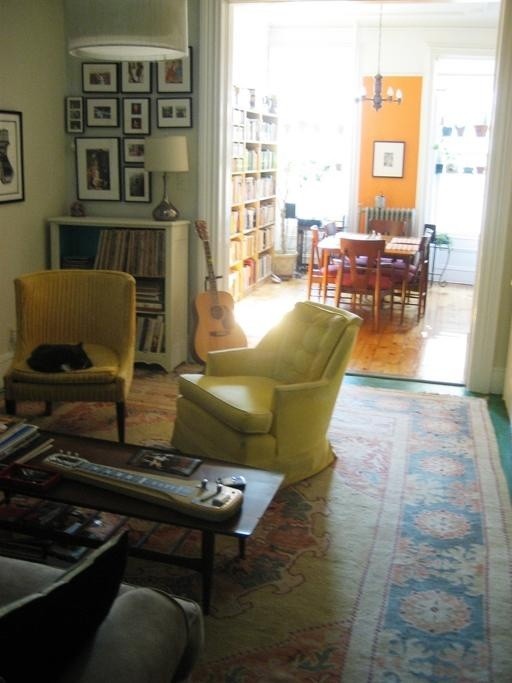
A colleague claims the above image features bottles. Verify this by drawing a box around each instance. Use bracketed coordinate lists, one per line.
[378, 195, 385, 209]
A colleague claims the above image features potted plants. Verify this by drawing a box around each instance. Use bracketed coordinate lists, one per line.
[270, 162, 342, 280]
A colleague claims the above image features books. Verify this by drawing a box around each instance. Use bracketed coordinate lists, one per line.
[1, 414, 53, 468]
[230, 86, 277, 298]
[65, 227, 165, 356]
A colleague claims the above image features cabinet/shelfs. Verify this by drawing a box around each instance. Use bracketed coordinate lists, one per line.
[47, 216, 192, 373]
[230, 85, 281, 300]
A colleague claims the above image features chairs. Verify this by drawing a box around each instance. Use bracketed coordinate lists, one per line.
[0, 556, 204, 683]
[3, 269, 136, 446]
[171, 301, 364, 489]
[304, 224, 433, 336]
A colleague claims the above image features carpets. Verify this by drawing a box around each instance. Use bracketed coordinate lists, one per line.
[1, 374, 511, 682]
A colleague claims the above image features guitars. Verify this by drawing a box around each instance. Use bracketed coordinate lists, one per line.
[190, 218, 246, 365]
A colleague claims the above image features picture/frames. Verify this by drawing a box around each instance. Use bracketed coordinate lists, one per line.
[371, 141, 405, 179]
[62, 46, 193, 201]
[0, 110, 25, 204]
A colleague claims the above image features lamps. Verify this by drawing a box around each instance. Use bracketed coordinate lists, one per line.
[354, 4, 403, 110]
[144, 136, 189, 220]
[63, 0, 188, 61]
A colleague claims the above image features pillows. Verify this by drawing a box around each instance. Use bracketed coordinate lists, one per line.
[1, 519, 125, 682]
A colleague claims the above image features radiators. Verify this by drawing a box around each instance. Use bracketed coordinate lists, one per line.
[354, 207, 415, 238]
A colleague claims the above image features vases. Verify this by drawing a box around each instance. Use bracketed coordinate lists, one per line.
[475, 125, 488, 137]
